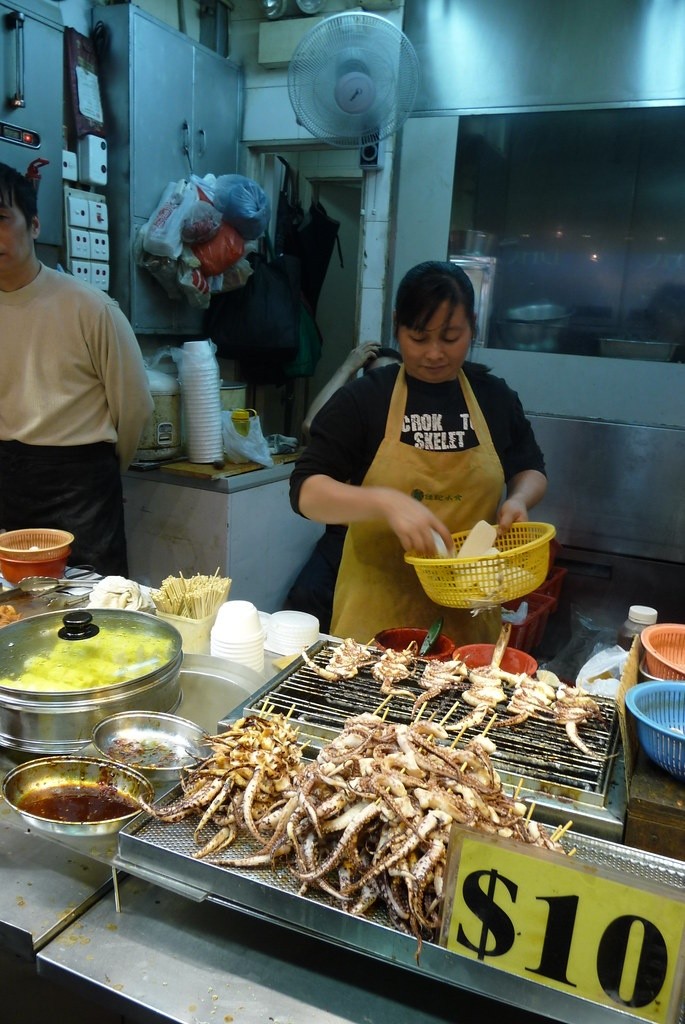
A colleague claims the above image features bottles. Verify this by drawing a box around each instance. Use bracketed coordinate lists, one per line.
[227, 408, 257, 463]
[617, 605, 658, 651]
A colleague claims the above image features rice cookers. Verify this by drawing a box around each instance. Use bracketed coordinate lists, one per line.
[129, 369, 183, 460]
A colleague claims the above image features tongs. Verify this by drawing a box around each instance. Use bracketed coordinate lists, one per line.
[16, 574, 100, 612]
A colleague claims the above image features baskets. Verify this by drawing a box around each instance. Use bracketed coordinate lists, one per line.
[536, 565, 567, 615]
[640, 623, 685, 682]
[625, 679, 685, 779]
[499, 592, 557, 651]
[405, 521, 557, 610]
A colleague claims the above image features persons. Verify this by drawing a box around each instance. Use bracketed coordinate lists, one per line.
[0, 162, 155, 578]
[282, 340, 402, 636]
[289, 261, 549, 650]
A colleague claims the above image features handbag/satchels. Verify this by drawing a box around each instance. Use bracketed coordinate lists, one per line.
[133, 171, 299, 350]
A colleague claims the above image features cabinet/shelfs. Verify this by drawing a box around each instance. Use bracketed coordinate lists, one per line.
[91, 4, 246, 336]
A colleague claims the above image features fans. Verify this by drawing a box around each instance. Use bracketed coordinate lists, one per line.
[287, 10, 422, 172]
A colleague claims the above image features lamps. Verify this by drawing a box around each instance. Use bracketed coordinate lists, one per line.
[257, 0, 326, 71]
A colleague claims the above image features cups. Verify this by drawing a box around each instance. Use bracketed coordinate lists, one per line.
[465, 228, 495, 257]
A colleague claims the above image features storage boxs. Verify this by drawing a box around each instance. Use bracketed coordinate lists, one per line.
[500, 591, 558, 653]
[534, 567, 568, 614]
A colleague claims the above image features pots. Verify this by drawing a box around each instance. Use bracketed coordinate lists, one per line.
[0, 606, 184, 754]
[220, 381, 247, 411]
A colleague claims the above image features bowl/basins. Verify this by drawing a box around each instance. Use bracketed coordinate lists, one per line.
[0, 755, 155, 837]
[210, 600, 320, 673]
[181, 341, 225, 464]
[599, 336, 680, 362]
[0, 547, 71, 584]
[503, 302, 573, 326]
[499, 320, 569, 354]
[90, 710, 214, 782]
[373, 627, 455, 662]
[452, 643, 538, 676]
